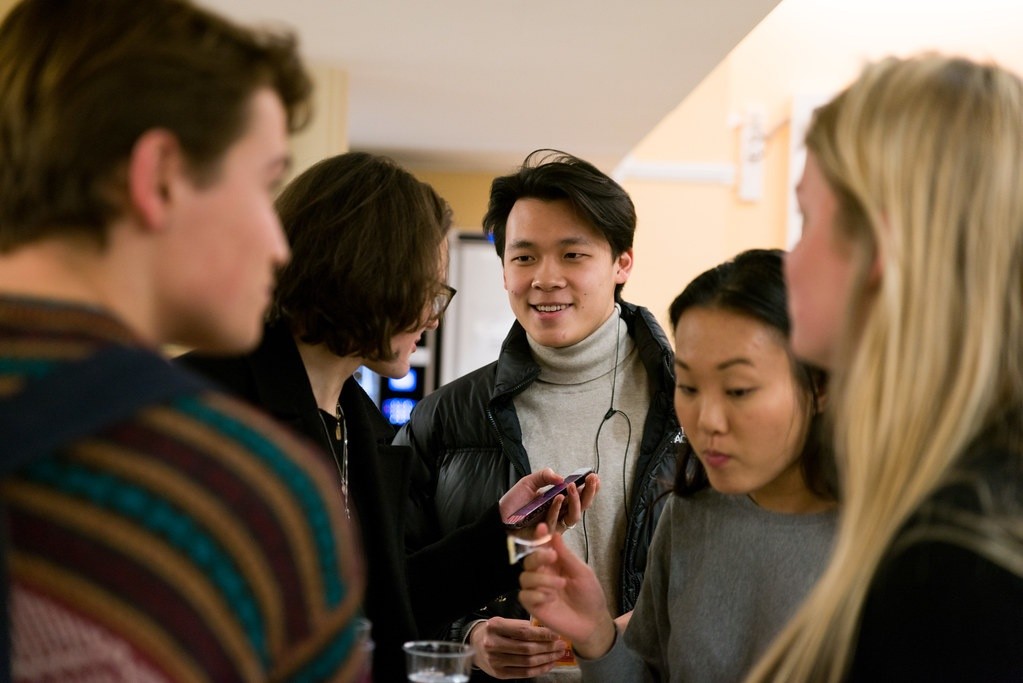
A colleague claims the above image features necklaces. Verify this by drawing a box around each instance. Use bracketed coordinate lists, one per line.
[313, 391, 351, 521]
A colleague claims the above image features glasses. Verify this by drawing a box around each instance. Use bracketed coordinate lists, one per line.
[425, 276, 457, 319]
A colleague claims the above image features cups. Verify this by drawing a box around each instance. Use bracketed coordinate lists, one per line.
[403, 640, 477, 683]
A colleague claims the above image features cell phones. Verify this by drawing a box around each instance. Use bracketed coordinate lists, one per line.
[504, 466, 597, 530]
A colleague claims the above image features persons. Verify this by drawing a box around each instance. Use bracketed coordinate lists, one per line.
[743, 49, 1023, 682]
[1, 1, 372, 682]
[169, 145, 600, 683]
[516, 247, 843, 682]
[388, 150, 697, 683]
